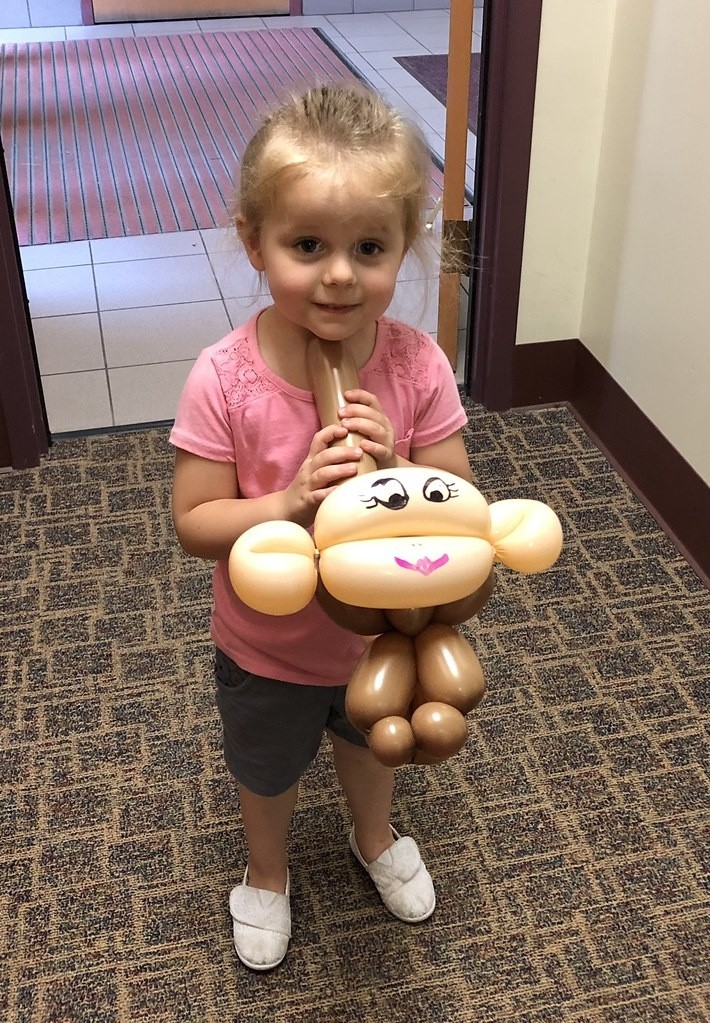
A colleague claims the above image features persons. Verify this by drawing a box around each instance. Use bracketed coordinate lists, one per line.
[166, 84, 475, 970]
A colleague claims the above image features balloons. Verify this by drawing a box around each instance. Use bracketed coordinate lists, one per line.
[229, 336, 562, 767]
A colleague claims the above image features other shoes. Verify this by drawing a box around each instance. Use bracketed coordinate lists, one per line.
[228, 858, 291, 970]
[348, 817, 435, 922]
